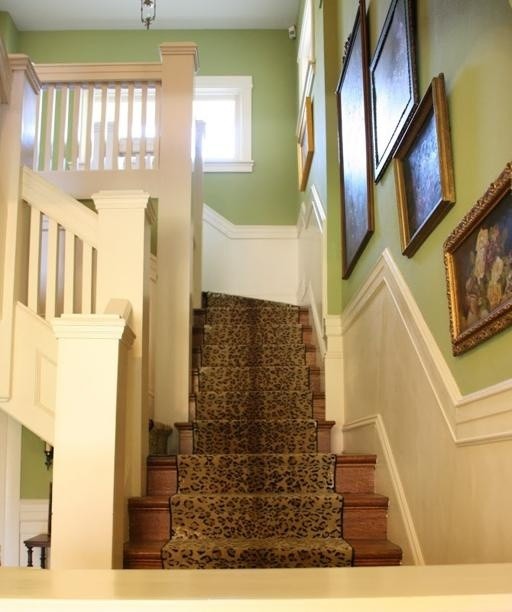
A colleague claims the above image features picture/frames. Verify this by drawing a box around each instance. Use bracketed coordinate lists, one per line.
[368, 0, 421, 185]
[390, 72, 457, 259]
[295, 93, 316, 194]
[334, 0, 378, 281]
[439, 158, 512, 357]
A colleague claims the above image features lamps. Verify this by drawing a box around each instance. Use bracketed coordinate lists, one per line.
[140, 0, 157, 31]
[44, 441, 54, 471]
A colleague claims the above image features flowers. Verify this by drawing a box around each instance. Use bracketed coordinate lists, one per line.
[465, 221, 512, 320]
